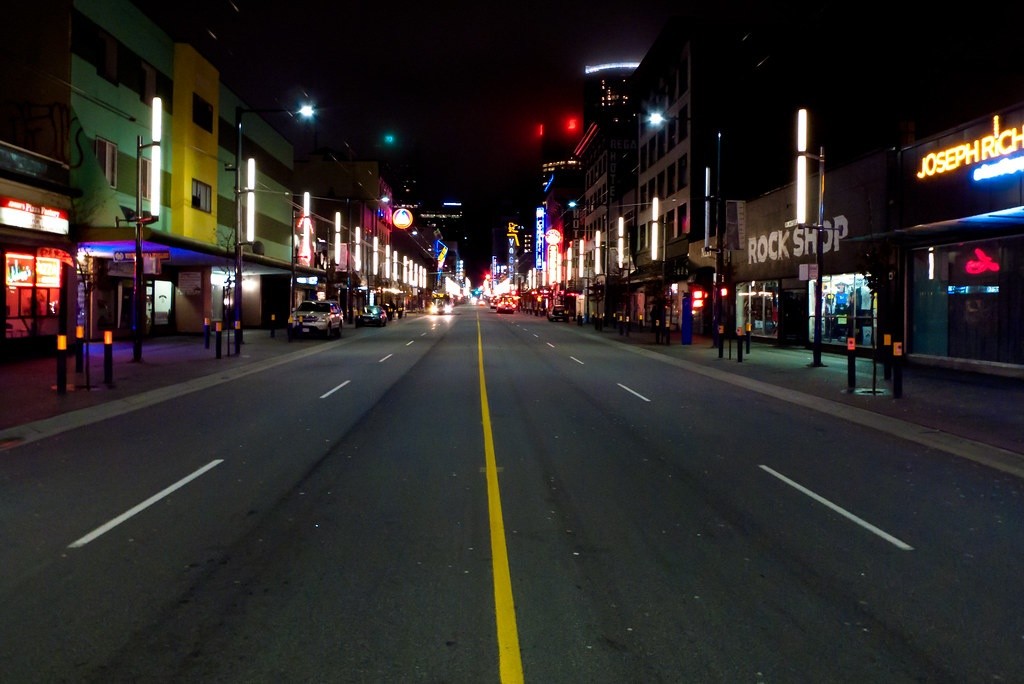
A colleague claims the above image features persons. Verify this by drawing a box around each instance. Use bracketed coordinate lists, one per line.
[380, 297, 408, 322]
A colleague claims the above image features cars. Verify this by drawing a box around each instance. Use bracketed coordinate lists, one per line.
[547, 305, 570, 323]
[496, 303, 514, 314]
[355, 305, 387, 328]
[287, 300, 342, 339]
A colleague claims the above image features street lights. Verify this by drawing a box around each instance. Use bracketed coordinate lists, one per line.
[702, 130, 725, 345]
[576, 237, 590, 323]
[617, 216, 631, 333]
[649, 195, 670, 295]
[134, 95, 164, 361]
[230, 104, 257, 345]
[792, 107, 827, 367]
